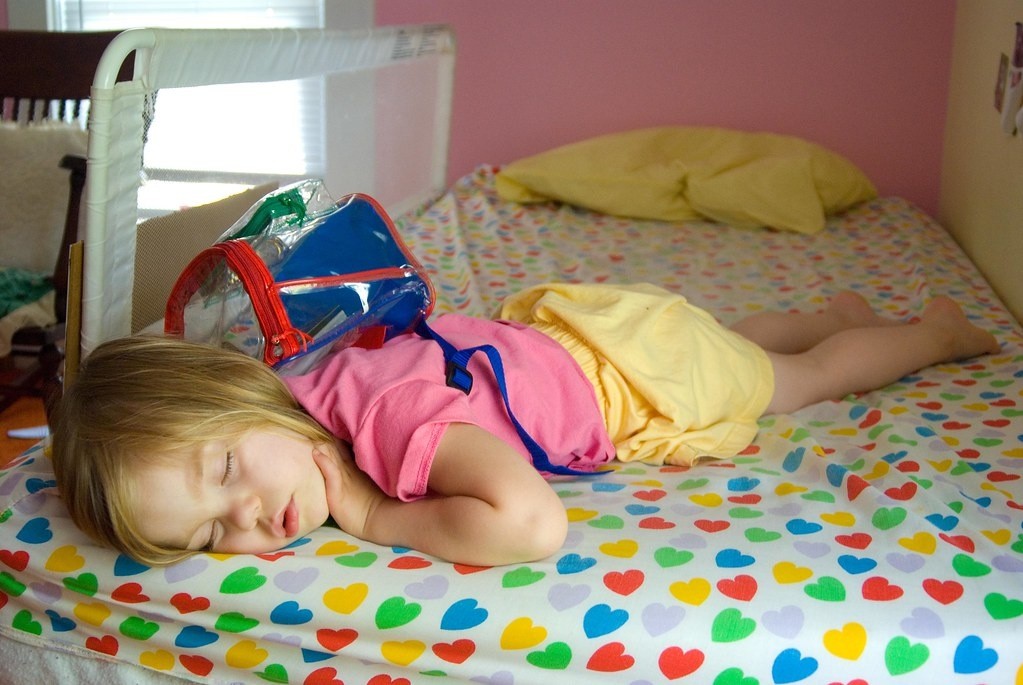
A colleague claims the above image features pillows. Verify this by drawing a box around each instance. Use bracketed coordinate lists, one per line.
[495, 123, 879, 235]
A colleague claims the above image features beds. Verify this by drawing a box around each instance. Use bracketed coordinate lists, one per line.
[0, 25, 1023, 685]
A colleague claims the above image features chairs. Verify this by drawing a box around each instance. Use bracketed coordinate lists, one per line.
[0, 30, 157, 434]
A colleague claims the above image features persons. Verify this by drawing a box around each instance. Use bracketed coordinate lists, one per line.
[50, 281, 1002, 567]
